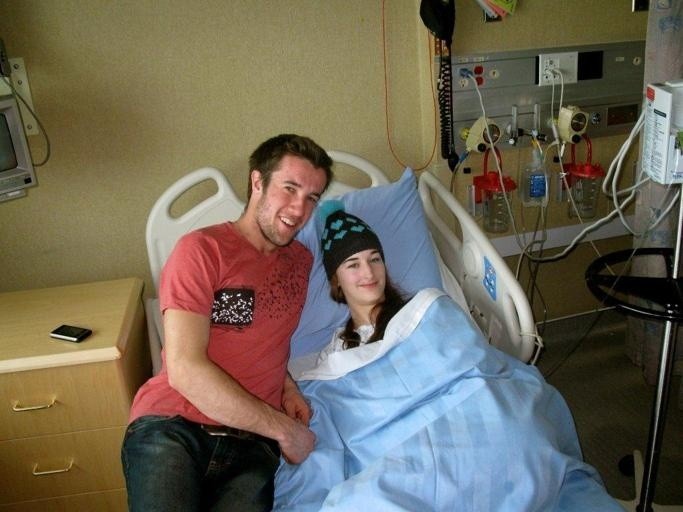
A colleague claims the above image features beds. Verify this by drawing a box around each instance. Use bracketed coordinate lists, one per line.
[141, 149, 627, 512]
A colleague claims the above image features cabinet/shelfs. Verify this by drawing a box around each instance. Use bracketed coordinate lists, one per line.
[0, 276, 145, 512]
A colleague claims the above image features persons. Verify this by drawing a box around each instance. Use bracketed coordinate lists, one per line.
[315, 211, 420, 357]
[121, 134, 333, 511]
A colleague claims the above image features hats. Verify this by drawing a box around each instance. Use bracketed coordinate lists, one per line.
[320, 209, 384, 281]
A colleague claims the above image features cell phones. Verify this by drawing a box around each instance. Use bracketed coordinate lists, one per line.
[49, 323, 92, 343]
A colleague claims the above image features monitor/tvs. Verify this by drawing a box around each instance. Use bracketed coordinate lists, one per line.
[1, 92, 39, 204]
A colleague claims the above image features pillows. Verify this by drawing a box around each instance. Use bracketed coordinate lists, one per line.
[286, 166, 446, 360]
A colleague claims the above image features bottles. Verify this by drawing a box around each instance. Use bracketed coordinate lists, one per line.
[567, 161, 604, 219]
[519, 150, 550, 209]
[472, 171, 516, 235]
